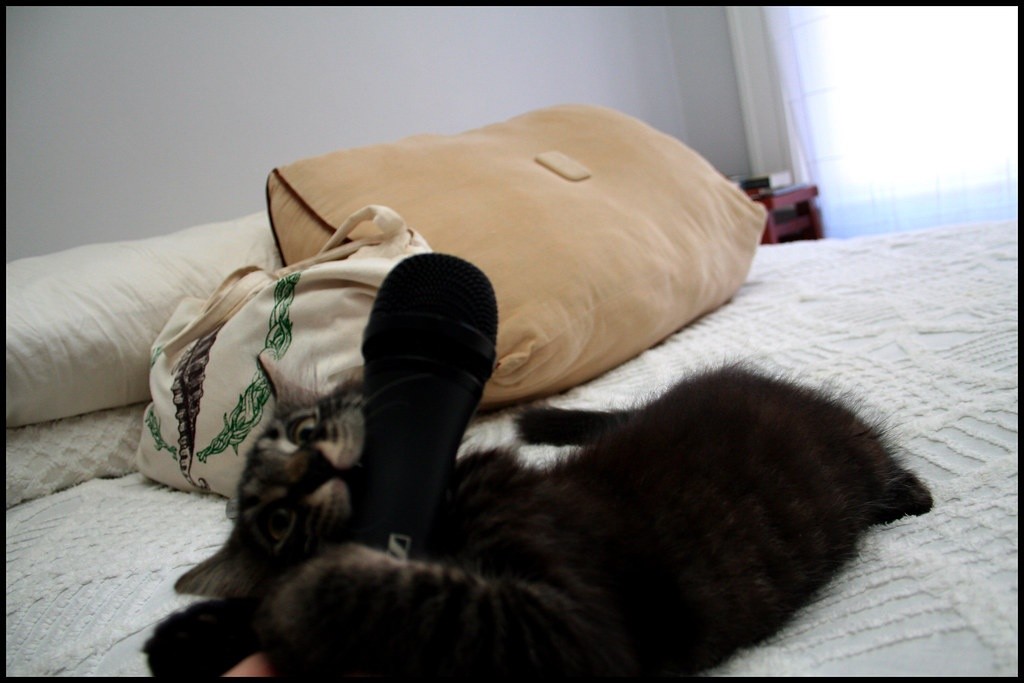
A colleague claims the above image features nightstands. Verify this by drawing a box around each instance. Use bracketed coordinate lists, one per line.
[752, 185, 825, 245]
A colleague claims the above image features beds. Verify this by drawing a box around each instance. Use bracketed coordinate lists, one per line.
[7, 210, 1020, 678]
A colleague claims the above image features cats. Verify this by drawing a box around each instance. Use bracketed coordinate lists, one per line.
[173, 252, 935, 678]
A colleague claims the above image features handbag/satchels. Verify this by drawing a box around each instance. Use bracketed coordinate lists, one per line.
[137, 205, 434, 499]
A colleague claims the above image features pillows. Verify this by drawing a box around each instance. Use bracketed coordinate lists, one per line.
[266, 103, 770, 410]
